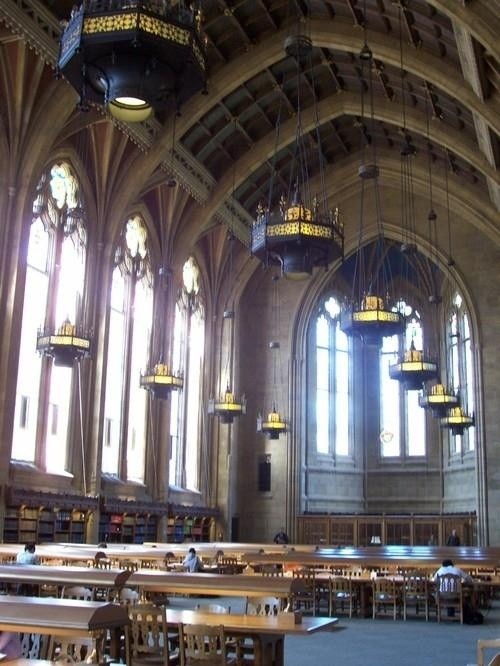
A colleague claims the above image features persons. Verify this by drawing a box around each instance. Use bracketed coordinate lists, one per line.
[17, 543, 40, 565]
[446, 529, 460, 546]
[273, 527, 289, 544]
[432, 559, 473, 599]
[182, 547, 204, 572]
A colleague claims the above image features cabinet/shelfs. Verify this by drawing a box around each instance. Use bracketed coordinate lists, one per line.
[5, 503, 87, 543]
[99, 511, 157, 544]
[168, 516, 210, 544]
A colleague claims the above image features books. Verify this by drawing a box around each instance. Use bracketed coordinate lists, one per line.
[4, 506, 203, 544]
